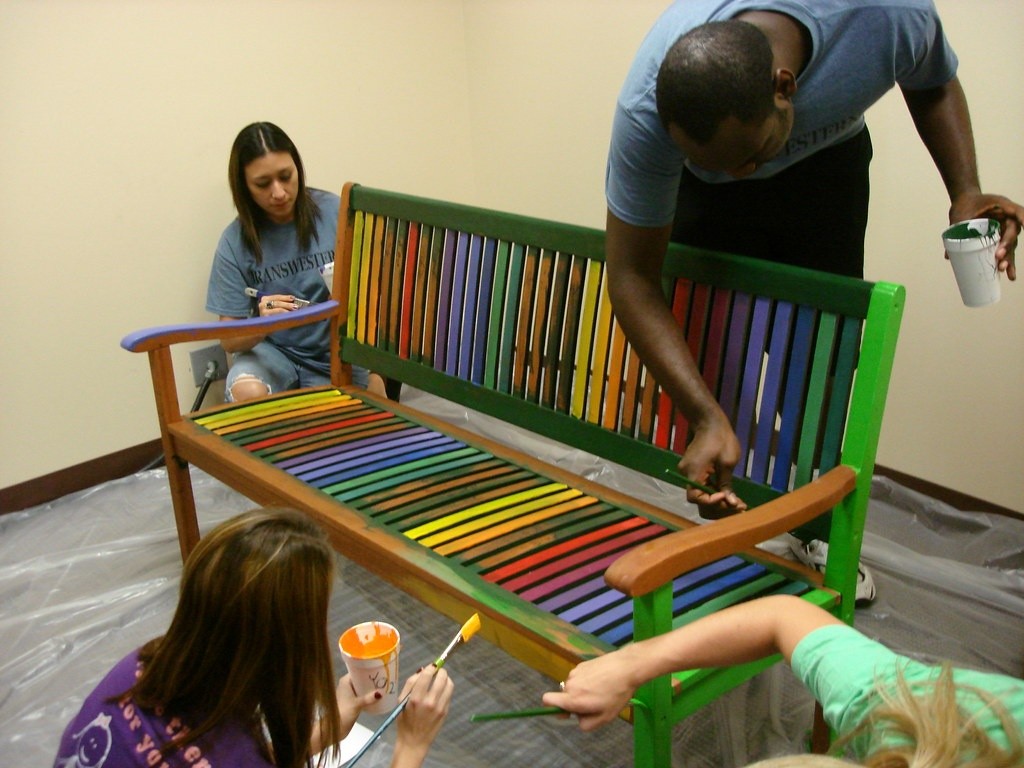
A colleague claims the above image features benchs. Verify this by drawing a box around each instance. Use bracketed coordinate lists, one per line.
[120, 182, 906, 768]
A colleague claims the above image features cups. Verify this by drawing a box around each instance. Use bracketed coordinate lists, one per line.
[338, 621, 400, 714]
[941, 218, 1002, 308]
[318, 261, 334, 297]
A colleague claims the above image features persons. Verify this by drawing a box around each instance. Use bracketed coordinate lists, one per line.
[206, 121, 389, 403]
[52, 507, 454, 768]
[605, 0, 1024, 608]
[544, 594, 1024, 768]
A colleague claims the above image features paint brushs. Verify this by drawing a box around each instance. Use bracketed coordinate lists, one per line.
[247, 288, 310, 309]
[664, 467, 729, 505]
[342, 612, 485, 768]
[465, 704, 565, 728]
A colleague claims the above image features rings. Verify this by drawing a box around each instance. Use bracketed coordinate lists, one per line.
[266, 300, 274, 309]
